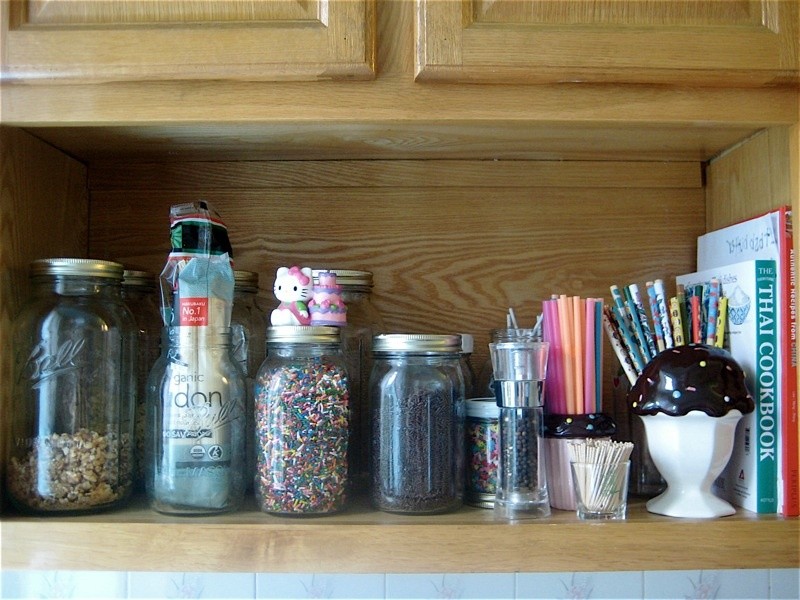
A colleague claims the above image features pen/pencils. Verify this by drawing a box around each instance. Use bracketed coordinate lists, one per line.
[695, 285, 703, 302]
[646, 281, 665, 352]
[654, 279, 672, 349]
[706, 280, 720, 345]
[676, 284, 692, 346]
[701, 283, 710, 345]
[629, 284, 657, 357]
[691, 296, 702, 345]
[603, 304, 644, 375]
[610, 284, 639, 352]
[623, 286, 652, 364]
[715, 296, 727, 349]
[602, 315, 638, 385]
[668, 296, 685, 349]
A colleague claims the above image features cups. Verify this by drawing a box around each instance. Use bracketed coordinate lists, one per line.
[543, 413, 616, 510]
[570, 460, 631, 520]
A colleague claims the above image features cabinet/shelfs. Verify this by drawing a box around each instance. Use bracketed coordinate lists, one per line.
[0, 0, 799, 570]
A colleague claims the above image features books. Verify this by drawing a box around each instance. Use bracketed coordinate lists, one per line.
[676, 205, 800, 516]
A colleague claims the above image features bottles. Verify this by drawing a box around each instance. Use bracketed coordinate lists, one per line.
[254, 327, 350, 516]
[365, 326, 552, 518]
[311, 269, 386, 491]
[120, 269, 160, 487]
[231, 269, 267, 484]
[9, 258, 139, 511]
[145, 202, 247, 517]
